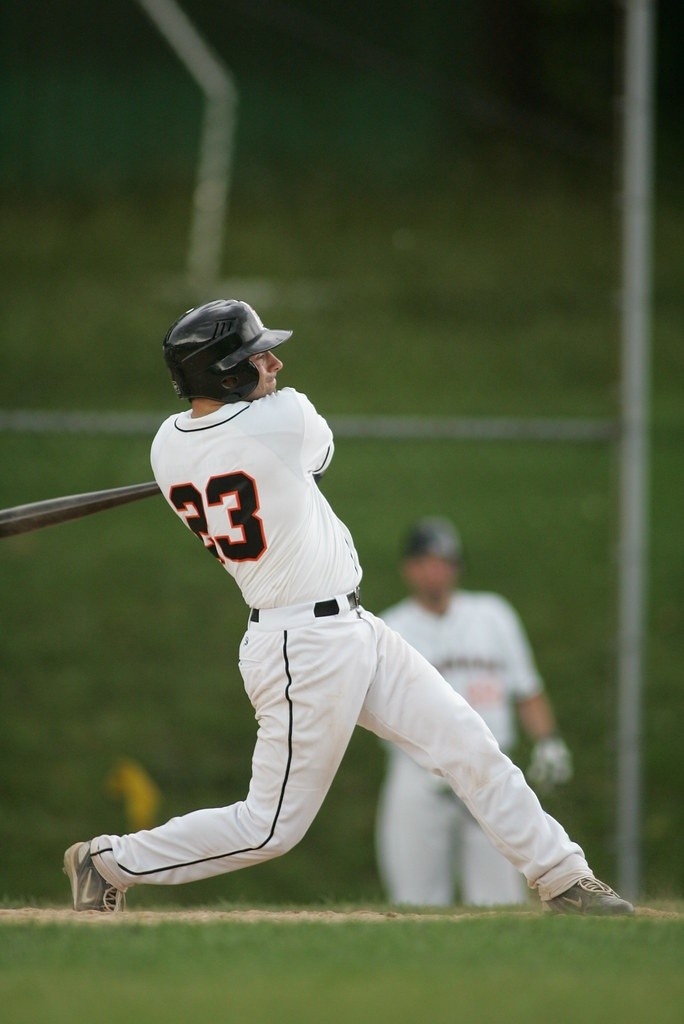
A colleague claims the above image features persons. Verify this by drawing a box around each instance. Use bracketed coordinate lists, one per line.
[372, 515, 575, 906]
[62, 299, 635, 915]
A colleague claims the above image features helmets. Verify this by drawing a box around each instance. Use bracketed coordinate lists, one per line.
[398, 515, 461, 568]
[161, 299, 294, 403]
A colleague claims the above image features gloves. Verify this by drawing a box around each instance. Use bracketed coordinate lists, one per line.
[527, 736, 574, 786]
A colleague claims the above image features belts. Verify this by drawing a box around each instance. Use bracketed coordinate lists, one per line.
[251, 589, 360, 622]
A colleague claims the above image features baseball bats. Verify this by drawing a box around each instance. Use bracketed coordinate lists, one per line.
[0, 477, 162, 540]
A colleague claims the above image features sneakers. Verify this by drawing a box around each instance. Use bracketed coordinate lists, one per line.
[542, 877, 634, 916]
[64, 840, 126, 912]
